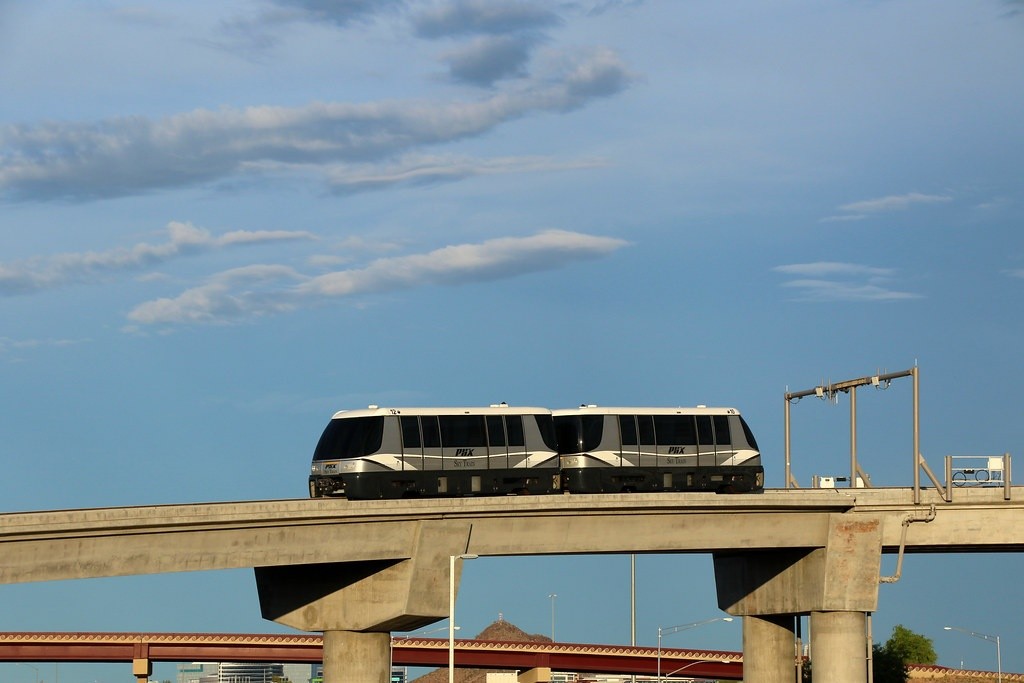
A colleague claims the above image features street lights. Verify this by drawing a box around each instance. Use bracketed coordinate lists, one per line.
[448, 554, 479, 683]
[390, 626, 461, 683]
[548, 594, 558, 643]
[658, 618, 735, 683]
[943, 625, 1002, 683]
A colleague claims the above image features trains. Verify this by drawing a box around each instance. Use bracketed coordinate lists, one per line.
[309, 403, 765, 500]
